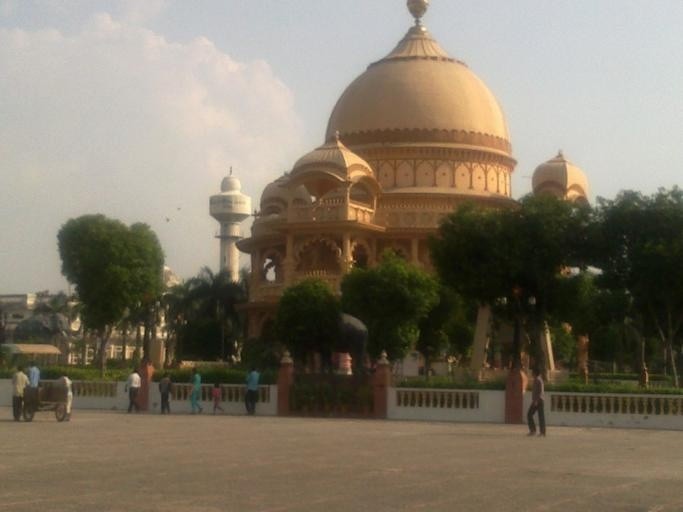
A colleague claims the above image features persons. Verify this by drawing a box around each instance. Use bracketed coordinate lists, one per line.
[159, 372, 172, 414]
[29, 360, 40, 387]
[212, 383, 225, 416]
[190, 367, 203, 414]
[527, 368, 546, 437]
[125, 366, 142, 413]
[12, 366, 30, 422]
[58, 371, 74, 421]
[244, 364, 260, 415]
[642, 367, 649, 384]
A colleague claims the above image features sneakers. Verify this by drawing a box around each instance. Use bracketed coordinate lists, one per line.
[526, 428, 545, 438]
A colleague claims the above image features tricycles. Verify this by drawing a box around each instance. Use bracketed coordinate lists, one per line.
[22, 379, 69, 422]
